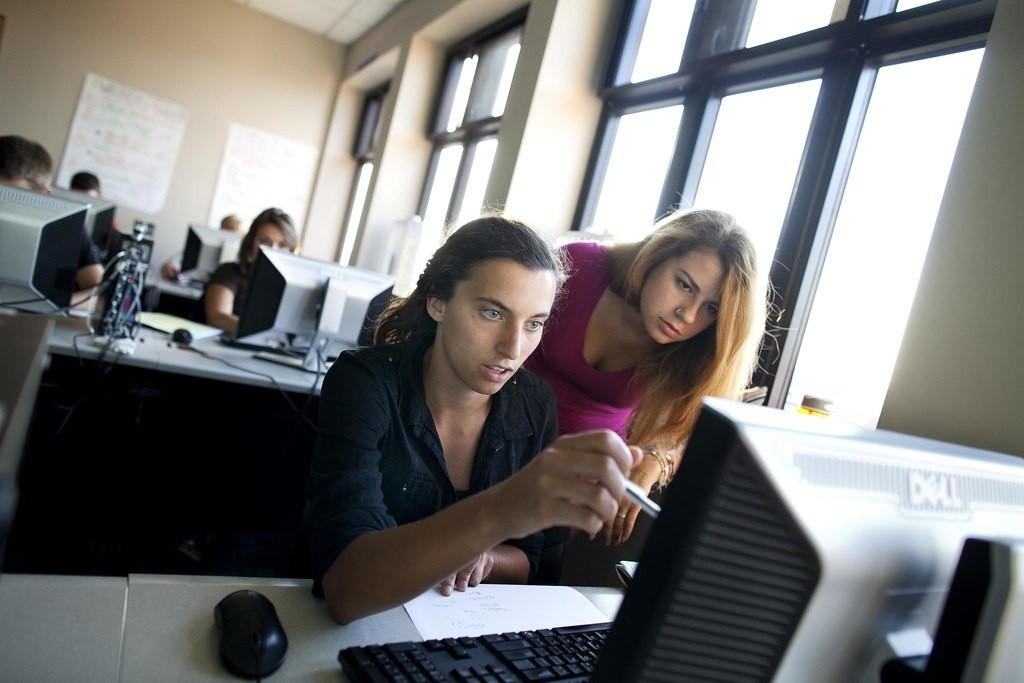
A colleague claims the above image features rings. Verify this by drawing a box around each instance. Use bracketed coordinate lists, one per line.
[618, 513, 626, 519]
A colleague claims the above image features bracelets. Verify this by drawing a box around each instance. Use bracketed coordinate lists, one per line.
[648, 448, 674, 486]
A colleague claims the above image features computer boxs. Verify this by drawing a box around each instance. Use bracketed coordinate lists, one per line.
[88, 219, 155, 337]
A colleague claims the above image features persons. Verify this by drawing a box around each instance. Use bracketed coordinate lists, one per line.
[69, 171, 122, 232]
[196, 208, 299, 332]
[0, 133, 105, 310]
[160, 214, 242, 286]
[297, 217, 644, 624]
[520, 208, 770, 586]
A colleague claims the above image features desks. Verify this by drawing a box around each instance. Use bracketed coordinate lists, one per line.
[47, 270, 356, 397]
[0, 570, 623, 683]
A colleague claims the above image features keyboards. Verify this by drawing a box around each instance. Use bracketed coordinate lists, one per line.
[220, 334, 306, 358]
[337, 621, 613, 683]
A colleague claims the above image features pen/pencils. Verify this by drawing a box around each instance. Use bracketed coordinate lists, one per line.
[621, 475, 663, 520]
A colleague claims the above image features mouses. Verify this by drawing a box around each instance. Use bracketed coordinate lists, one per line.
[214, 589, 288, 680]
[173, 329, 193, 345]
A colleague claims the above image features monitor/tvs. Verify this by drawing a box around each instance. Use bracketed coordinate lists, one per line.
[0, 181, 116, 318]
[587, 393, 1024, 683]
[230, 244, 397, 375]
[180, 224, 244, 285]
